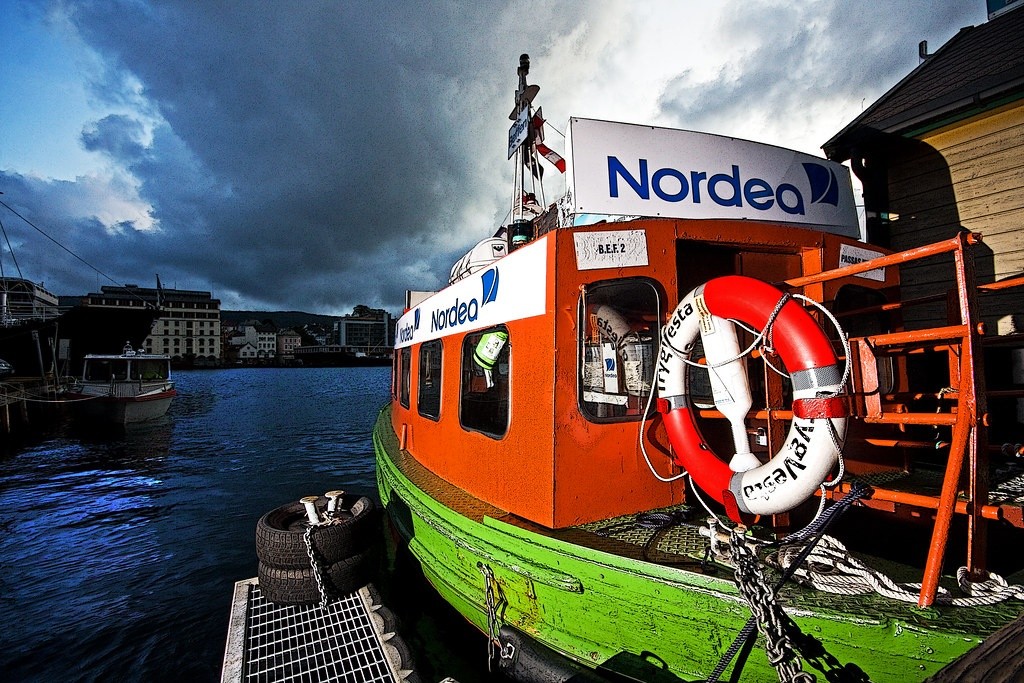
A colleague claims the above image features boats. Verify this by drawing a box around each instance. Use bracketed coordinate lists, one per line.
[68, 340, 177, 426]
[370, 51, 1024, 682]
[21, 386, 123, 437]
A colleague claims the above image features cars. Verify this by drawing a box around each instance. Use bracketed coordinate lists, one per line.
[0, 358, 13, 381]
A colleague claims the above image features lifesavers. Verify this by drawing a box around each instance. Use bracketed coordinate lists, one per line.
[582, 302, 645, 419]
[653, 273, 844, 519]
[252, 493, 385, 605]
